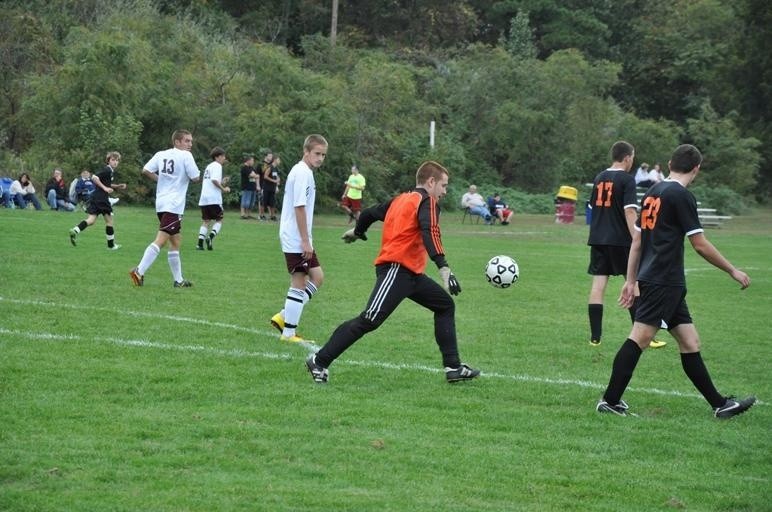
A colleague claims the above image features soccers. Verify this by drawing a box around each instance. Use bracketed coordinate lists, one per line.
[485, 254, 520, 289]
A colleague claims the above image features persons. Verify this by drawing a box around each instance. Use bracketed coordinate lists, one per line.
[588, 141, 670, 350]
[462, 182, 496, 226]
[130, 129, 200, 289]
[307, 160, 480, 386]
[270, 134, 328, 344]
[490, 192, 514, 226]
[0, 178, 9, 210]
[597, 144, 757, 418]
[44, 166, 75, 212]
[260, 156, 280, 221]
[69, 151, 126, 251]
[256, 153, 273, 219]
[240, 156, 261, 219]
[650, 163, 665, 183]
[197, 146, 231, 251]
[341, 165, 366, 226]
[634, 162, 654, 190]
[76, 167, 119, 211]
[10, 172, 42, 211]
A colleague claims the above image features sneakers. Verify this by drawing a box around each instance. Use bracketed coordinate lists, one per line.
[107, 244, 122, 250]
[348, 215, 356, 225]
[240, 216, 248, 220]
[501, 219, 509, 225]
[279, 335, 315, 345]
[447, 363, 480, 382]
[130, 267, 144, 287]
[258, 215, 267, 222]
[715, 396, 756, 419]
[69, 228, 77, 247]
[650, 340, 667, 348]
[206, 236, 213, 250]
[174, 280, 193, 288]
[590, 340, 601, 346]
[596, 399, 639, 419]
[247, 215, 256, 220]
[305, 353, 328, 385]
[269, 216, 278, 222]
[271, 313, 284, 334]
[198, 237, 204, 251]
[108, 198, 119, 206]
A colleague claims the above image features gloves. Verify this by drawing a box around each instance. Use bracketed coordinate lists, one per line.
[341, 228, 367, 244]
[439, 266, 462, 296]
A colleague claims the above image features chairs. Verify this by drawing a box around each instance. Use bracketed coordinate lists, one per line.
[463, 207, 480, 225]
